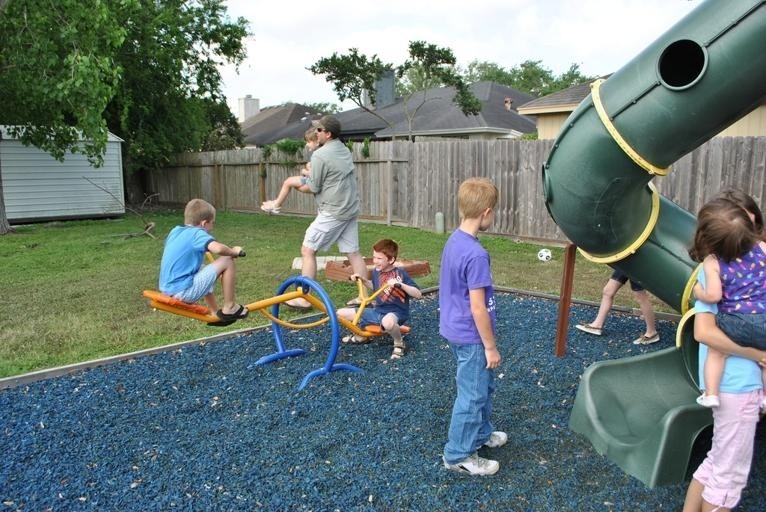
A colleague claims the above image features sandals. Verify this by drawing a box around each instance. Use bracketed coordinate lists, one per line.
[260, 200, 280, 213]
[391, 341, 405, 357]
[343, 334, 370, 345]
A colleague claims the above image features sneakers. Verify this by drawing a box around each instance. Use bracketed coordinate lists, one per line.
[575, 324, 602, 336]
[696, 393, 720, 408]
[475, 431, 507, 450]
[442, 453, 499, 475]
[633, 332, 660, 345]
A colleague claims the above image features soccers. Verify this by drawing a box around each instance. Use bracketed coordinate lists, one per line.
[538, 248, 552, 262]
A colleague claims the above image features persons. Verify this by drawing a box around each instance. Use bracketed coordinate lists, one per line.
[261, 127, 322, 214]
[438, 177, 508, 476]
[159, 198, 250, 327]
[687, 199, 766, 414]
[575, 271, 660, 344]
[682, 189, 766, 512]
[282, 115, 372, 312]
[336, 239, 422, 359]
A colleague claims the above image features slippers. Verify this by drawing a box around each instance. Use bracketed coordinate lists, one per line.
[345, 299, 372, 308]
[206, 304, 248, 326]
[282, 300, 312, 310]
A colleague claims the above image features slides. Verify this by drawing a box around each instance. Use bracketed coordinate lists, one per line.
[541, 0, 765, 488]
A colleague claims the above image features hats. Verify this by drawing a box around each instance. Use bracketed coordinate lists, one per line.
[311, 115, 339, 136]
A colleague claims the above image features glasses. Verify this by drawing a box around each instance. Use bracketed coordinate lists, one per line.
[317, 128, 325, 132]
